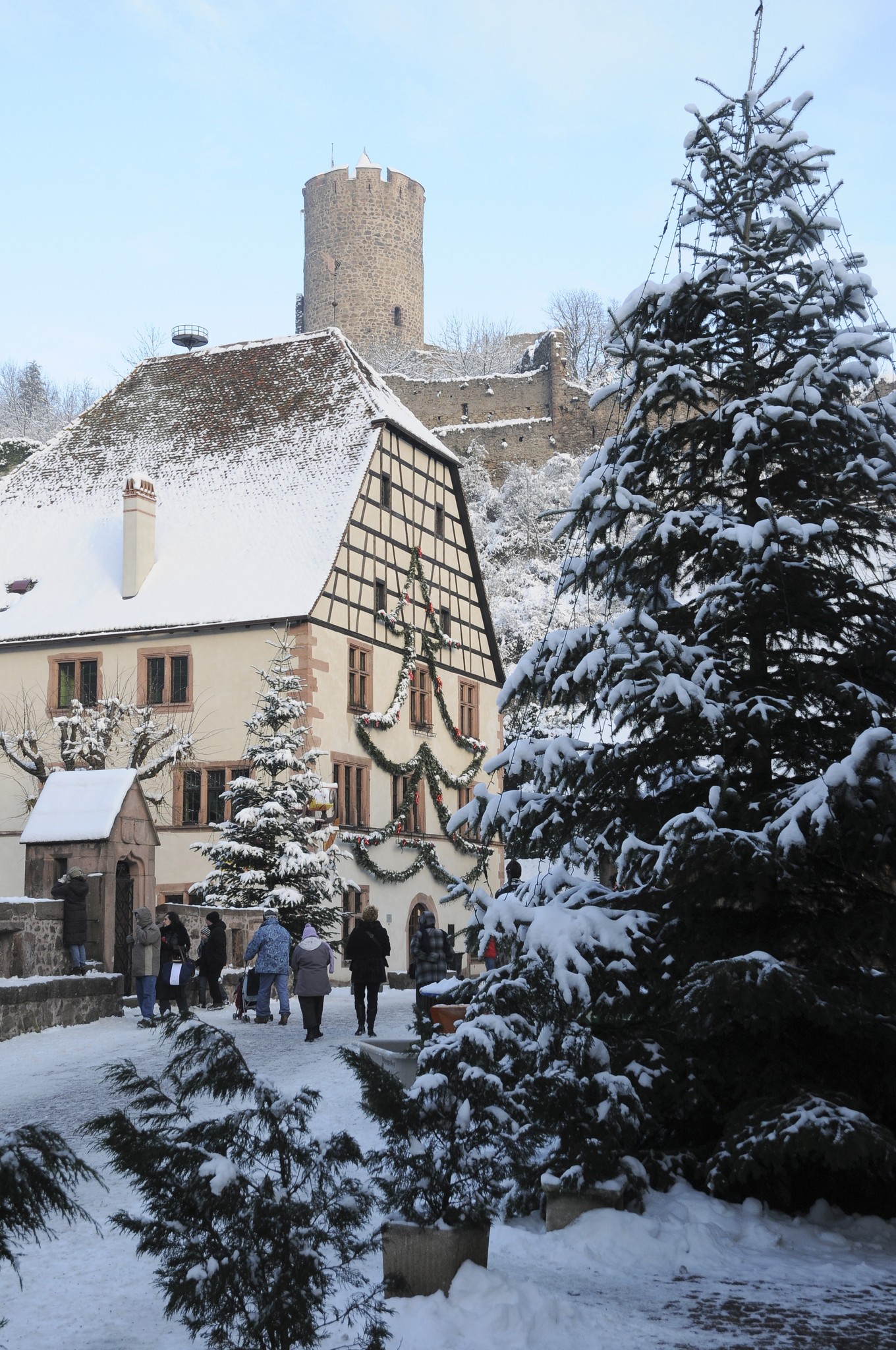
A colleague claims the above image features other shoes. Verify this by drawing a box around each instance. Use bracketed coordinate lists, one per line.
[305, 1027, 313, 1042]
[137, 1019, 157, 1028]
[81, 967, 87, 976]
[254, 1016, 267, 1024]
[314, 1028, 323, 1038]
[207, 1002, 224, 1011]
[279, 1014, 288, 1025]
[64, 969, 79, 976]
[355, 1024, 366, 1035]
[368, 1029, 376, 1037]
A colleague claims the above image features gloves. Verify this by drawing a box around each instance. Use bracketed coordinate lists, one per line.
[60, 876, 68, 883]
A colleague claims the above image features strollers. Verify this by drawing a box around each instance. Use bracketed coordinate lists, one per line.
[232, 961, 274, 1024]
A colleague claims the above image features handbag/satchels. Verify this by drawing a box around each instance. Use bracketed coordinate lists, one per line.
[161, 945, 193, 985]
[185, 956, 196, 977]
[407, 962, 417, 979]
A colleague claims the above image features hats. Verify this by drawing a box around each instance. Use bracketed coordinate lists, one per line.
[263, 909, 277, 921]
[201, 926, 211, 937]
[301, 923, 319, 941]
[69, 866, 81, 878]
[206, 911, 220, 924]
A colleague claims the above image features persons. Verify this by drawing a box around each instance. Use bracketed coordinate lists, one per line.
[158, 911, 191, 1016]
[409, 911, 455, 1016]
[343, 905, 391, 1037]
[291, 923, 334, 1041]
[194, 910, 229, 1011]
[50, 866, 90, 976]
[243, 910, 293, 1025]
[495, 860, 525, 899]
[126, 906, 161, 1026]
[485, 936, 498, 972]
